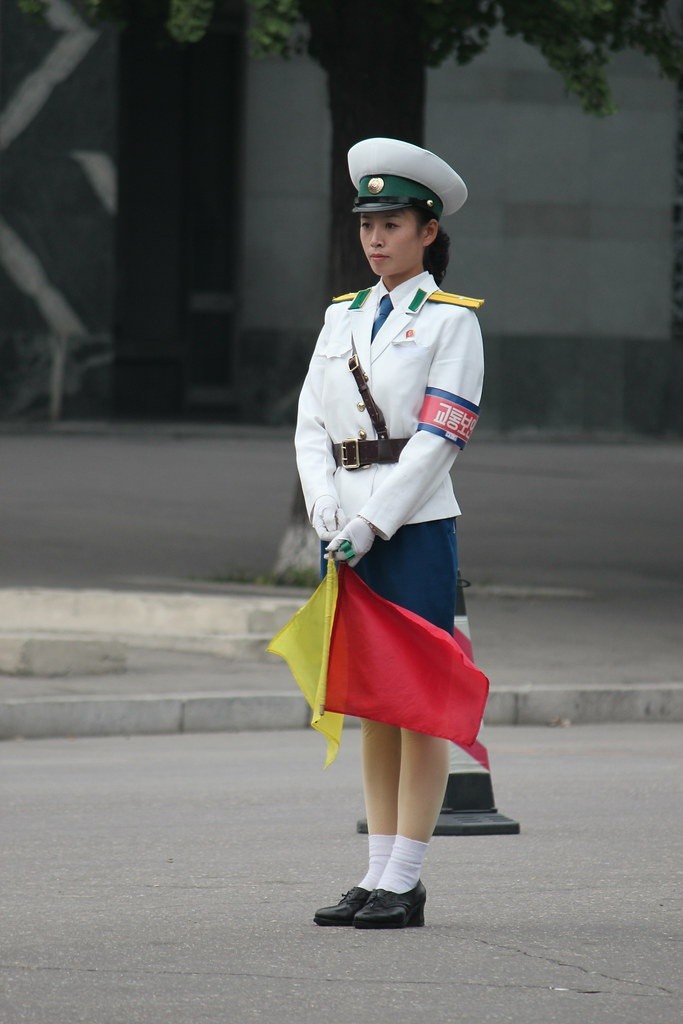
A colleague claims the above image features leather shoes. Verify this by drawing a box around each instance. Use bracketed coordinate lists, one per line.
[312, 887, 371, 926]
[354, 879, 426, 929]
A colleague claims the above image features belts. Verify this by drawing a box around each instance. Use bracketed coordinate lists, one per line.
[331, 439, 414, 471]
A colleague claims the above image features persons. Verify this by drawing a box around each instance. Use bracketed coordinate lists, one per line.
[289, 135, 488, 936]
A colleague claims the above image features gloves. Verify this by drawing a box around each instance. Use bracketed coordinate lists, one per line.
[310, 496, 344, 538]
[325, 517, 375, 566]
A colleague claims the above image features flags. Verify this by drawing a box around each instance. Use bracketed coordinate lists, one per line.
[264, 554, 350, 774]
[319, 562, 490, 749]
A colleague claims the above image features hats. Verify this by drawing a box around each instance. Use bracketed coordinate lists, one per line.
[343, 136, 468, 222]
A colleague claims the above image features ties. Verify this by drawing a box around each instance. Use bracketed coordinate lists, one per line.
[370, 297, 393, 346]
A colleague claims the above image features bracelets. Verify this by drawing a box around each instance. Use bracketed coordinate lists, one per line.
[358, 515, 381, 538]
